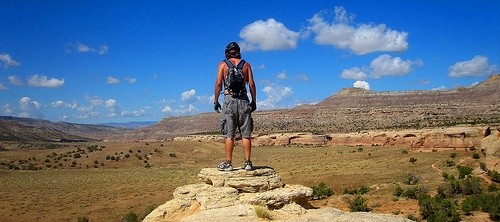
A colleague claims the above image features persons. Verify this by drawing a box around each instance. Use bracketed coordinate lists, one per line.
[213, 42, 257, 172]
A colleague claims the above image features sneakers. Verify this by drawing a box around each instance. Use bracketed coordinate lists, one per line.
[217, 160, 253, 171]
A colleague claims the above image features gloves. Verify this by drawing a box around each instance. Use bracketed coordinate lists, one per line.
[213, 101, 222, 113]
[249, 102, 257, 113]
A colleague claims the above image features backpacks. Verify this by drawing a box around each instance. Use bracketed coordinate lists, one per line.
[224, 59, 246, 97]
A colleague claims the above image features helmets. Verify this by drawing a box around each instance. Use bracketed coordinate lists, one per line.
[225, 41, 241, 58]
